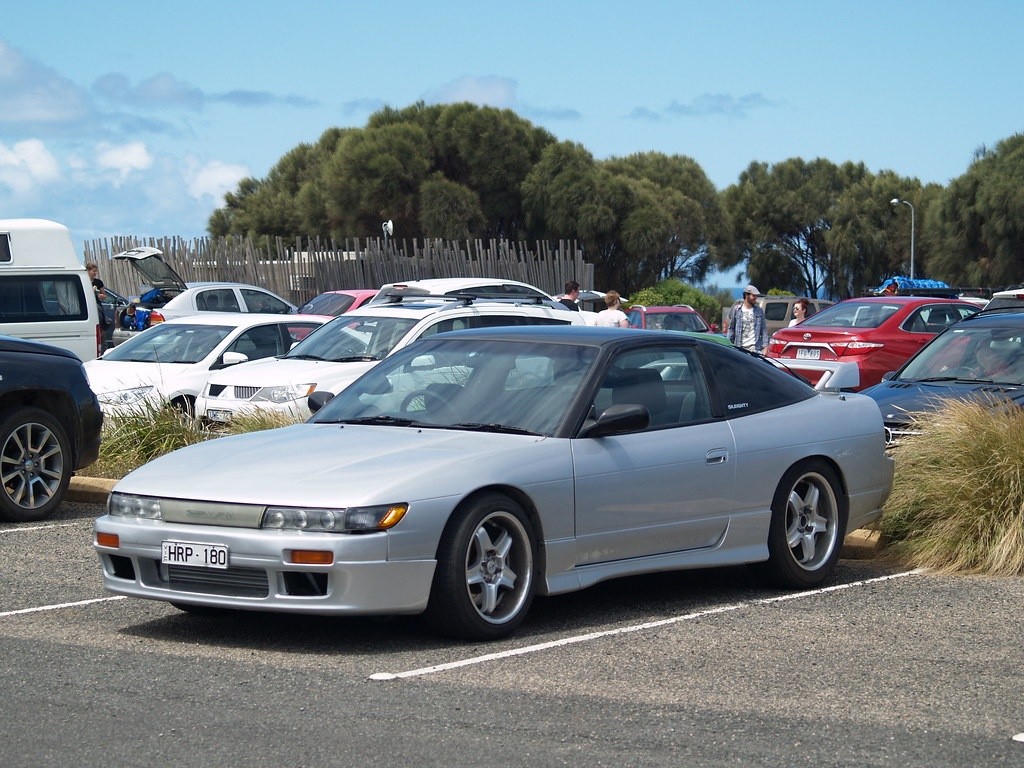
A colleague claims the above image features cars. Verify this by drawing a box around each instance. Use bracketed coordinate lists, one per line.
[194, 276, 630, 433]
[96, 286, 131, 331]
[722, 288, 1024, 398]
[854, 306, 1024, 442]
[723, 297, 838, 342]
[110, 245, 299, 349]
[551, 289, 629, 313]
[81, 313, 371, 437]
[90, 322, 897, 643]
[0, 333, 104, 522]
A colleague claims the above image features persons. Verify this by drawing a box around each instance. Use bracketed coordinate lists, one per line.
[789, 298, 810, 327]
[87, 263, 107, 330]
[558, 281, 581, 311]
[727, 285, 768, 355]
[593, 291, 628, 327]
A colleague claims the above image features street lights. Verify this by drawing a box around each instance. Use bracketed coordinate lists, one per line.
[889, 198, 915, 279]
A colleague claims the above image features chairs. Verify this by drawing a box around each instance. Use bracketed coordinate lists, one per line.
[424, 383, 475, 422]
[206, 294, 220, 311]
[991, 340, 1024, 365]
[191, 329, 223, 361]
[611, 367, 672, 425]
[250, 328, 277, 361]
[924, 309, 948, 333]
[221, 294, 238, 312]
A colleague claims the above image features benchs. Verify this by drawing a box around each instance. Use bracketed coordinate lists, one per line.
[592, 388, 700, 423]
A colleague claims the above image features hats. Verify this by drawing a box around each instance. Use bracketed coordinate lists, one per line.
[744, 285, 761, 296]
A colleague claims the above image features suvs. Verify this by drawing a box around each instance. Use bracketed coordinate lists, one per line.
[623, 303, 716, 335]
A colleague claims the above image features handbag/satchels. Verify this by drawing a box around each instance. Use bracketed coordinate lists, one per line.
[135, 306, 151, 331]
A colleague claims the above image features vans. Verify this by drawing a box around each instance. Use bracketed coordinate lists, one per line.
[0, 215, 104, 364]
